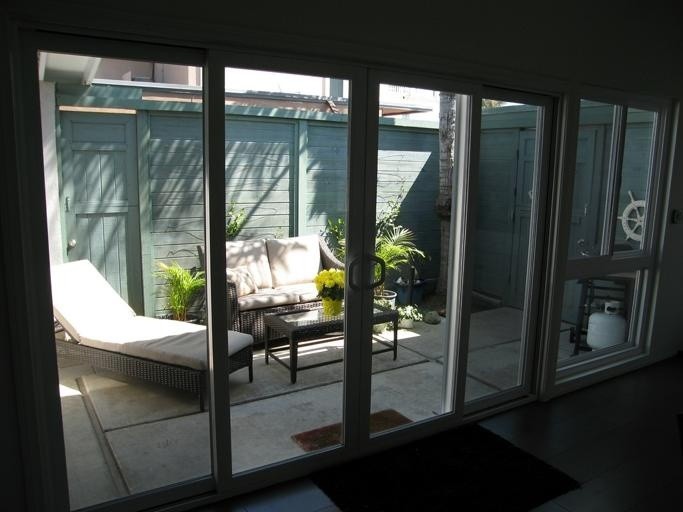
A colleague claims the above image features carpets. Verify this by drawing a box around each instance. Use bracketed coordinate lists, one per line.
[305, 423, 579, 512]
[291, 408, 413, 453]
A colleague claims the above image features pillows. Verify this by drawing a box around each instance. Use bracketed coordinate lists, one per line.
[225, 237, 273, 298]
[225, 265, 258, 296]
[266, 236, 324, 288]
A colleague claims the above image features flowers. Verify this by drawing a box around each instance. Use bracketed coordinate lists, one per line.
[314, 267, 345, 299]
[397, 303, 423, 322]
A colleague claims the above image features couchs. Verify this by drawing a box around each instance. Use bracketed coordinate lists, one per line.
[198, 237, 345, 346]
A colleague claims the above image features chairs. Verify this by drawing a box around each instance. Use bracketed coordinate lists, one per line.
[50, 259, 254, 411]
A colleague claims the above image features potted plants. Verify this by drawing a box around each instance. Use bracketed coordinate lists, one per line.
[372, 298, 391, 333]
[373, 225, 425, 307]
[152, 261, 206, 323]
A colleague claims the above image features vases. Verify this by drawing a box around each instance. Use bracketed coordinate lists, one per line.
[401, 318, 413, 328]
[322, 296, 342, 316]
